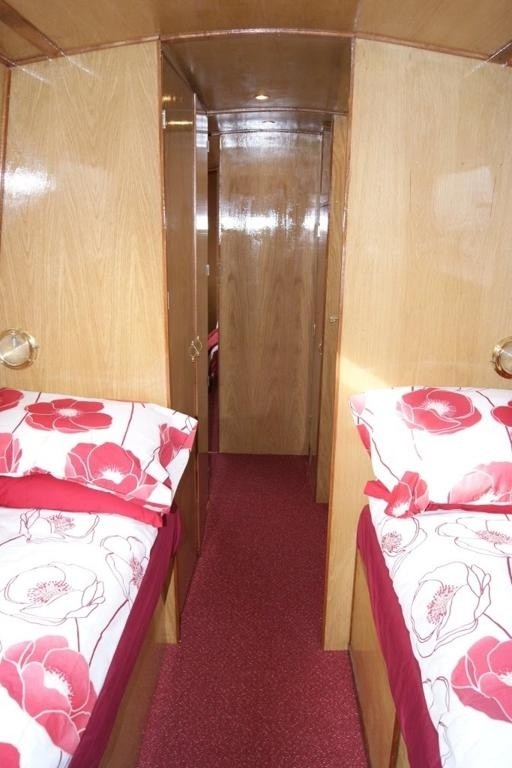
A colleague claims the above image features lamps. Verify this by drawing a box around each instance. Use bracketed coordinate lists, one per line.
[494, 337, 512, 380]
[1, 329, 39, 369]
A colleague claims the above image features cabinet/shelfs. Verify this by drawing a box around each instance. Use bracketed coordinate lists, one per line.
[2, 35, 211, 644]
[323, 41, 507, 656]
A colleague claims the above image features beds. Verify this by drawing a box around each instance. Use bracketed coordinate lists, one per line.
[348, 490, 512, 767]
[0, 500, 182, 768]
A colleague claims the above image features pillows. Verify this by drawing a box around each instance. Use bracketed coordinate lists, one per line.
[1, 387, 200, 515]
[1, 474, 164, 528]
[350, 385, 511, 518]
